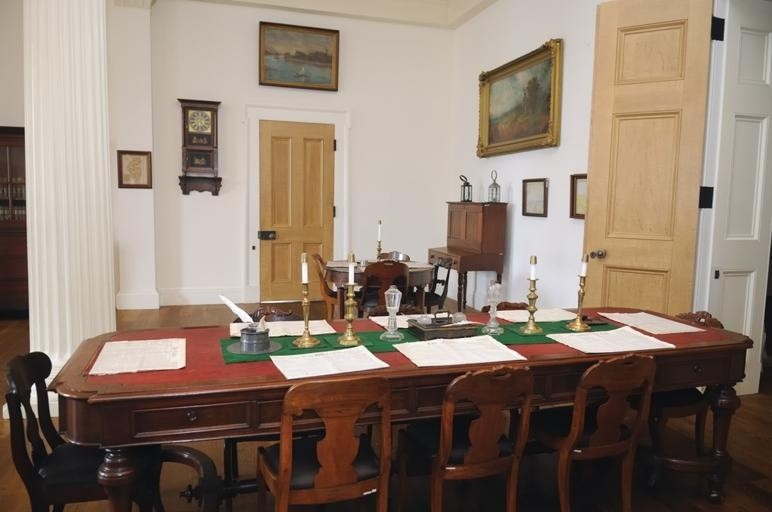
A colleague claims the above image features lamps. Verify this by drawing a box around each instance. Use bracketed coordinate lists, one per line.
[459, 175, 472, 202]
[488, 169, 500, 203]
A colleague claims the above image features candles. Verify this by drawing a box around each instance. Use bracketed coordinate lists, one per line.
[349, 263, 354, 284]
[530, 264, 536, 279]
[378, 224, 381, 241]
[578, 262, 588, 277]
[302, 262, 308, 283]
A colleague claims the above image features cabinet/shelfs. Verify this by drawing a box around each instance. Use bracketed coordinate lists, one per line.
[1, 126, 29, 312]
[429, 201, 508, 312]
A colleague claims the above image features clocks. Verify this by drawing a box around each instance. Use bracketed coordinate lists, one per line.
[179, 99, 223, 196]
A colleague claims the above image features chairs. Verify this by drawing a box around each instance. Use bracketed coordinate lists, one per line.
[312, 254, 360, 319]
[223, 307, 304, 512]
[653, 311, 726, 457]
[481, 302, 530, 312]
[399, 365, 535, 512]
[257, 377, 392, 512]
[530, 354, 655, 511]
[358, 260, 410, 317]
[364, 305, 424, 318]
[382, 251, 410, 261]
[5, 351, 166, 511]
[410, 256, 453, 314]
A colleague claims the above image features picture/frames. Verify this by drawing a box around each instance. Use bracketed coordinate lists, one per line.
[522, 179, 548, 216]
[117, 150, 152, 189]
[570, 174, 588, 218]
[259, 21, 339, 91]
[477, 39, 563, 158]
